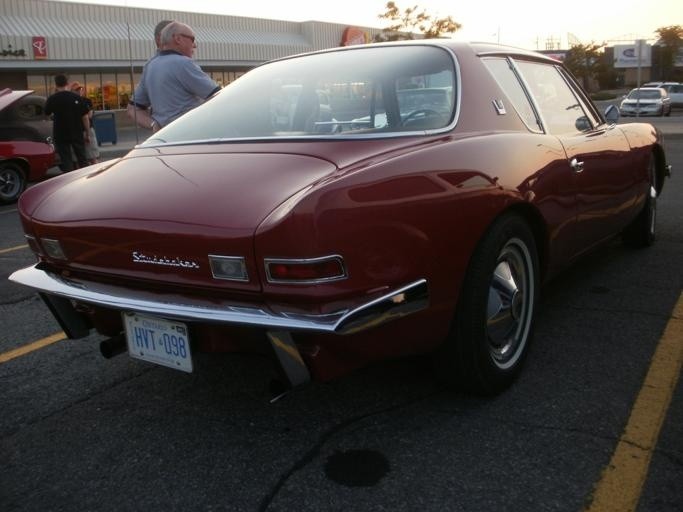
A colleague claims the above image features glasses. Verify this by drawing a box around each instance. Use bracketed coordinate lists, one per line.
[179, 33, 195, 43]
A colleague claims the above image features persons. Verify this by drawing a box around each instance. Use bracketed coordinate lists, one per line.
[143, 20, 178, 66]
[71, 81, 100, 167]
[45, 76, 90, 174]
[128, 21, 224, 134]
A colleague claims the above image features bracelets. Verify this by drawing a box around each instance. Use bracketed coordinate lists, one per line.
[151, 120, 157, 128]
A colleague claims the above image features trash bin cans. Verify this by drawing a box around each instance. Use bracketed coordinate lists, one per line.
[92, 111, 117, 146]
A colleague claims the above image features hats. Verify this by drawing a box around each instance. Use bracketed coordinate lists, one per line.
[69, 81, 84, 92]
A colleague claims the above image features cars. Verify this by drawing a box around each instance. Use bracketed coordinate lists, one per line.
[621, 86, 674, 119]
[642, 79, 683, 104]
[13, 39, 673, 395]
[2, 86, 57, 200]
